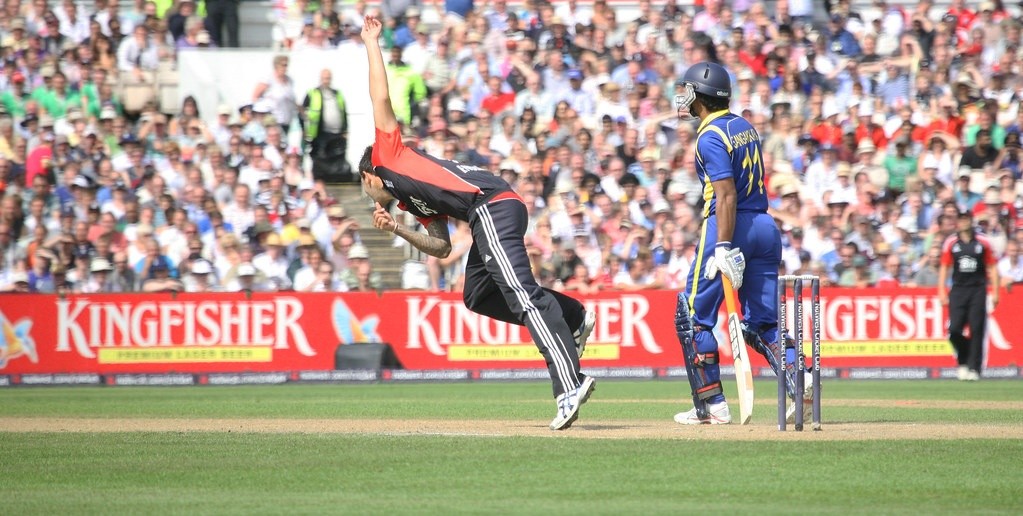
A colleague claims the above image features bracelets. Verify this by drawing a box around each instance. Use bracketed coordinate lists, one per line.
[392, 223, 398, 233]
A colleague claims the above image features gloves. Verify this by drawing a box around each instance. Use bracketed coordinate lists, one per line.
[704, 240, 746, 289]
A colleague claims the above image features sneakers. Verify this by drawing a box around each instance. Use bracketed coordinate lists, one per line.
[549, 375, 596, 430]
[673, 400, 732, 425]
[573, 310, 596, 357]
[786, 372, 824, 424]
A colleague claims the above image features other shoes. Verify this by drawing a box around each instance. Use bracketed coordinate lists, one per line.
[957, 363, 981, 380]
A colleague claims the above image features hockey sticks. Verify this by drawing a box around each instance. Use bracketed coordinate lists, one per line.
[722, 272, 754, 423]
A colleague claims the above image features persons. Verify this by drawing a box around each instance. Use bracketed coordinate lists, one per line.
[674, 60, 822, 427]
[0, 0, 1023, 294]
[359, 15, 597, 430]
[938, 208, 1000, 381]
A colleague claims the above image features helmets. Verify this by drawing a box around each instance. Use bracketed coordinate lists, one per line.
[682, 60, 732, 99]
